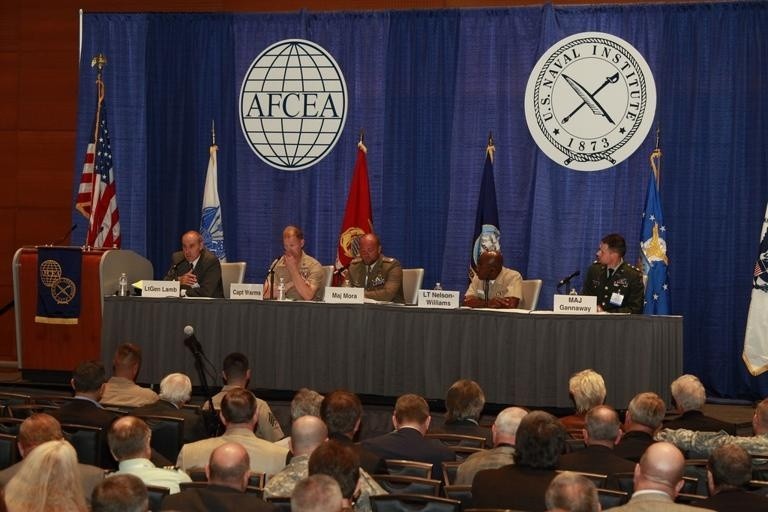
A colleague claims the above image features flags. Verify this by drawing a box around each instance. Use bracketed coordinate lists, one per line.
[638, 121, 672, 315]
[76, 53, 121, 249]
[468, 131, 503, 287]
[35, 251, 82, 324]
[332, 128, 374, 287]
[199, 120, 227, 263]
[741, 203, 768, 377]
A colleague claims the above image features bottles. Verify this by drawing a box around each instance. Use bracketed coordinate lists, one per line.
[434, 283, 442, 290]
[570, 288, 577, 295]
[118, 273, 127, 296]
[277, 278, 285, 300]
[344, 280, 350, 287]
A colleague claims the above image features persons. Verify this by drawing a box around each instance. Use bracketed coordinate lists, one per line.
[582, 233, 645, 314]
[318, 390, 388, 475]
[175, 388, 292, 486]
[0, 439, 88, 512]
[471, 410, 566, 512]
[170, 231, 224, 297]
[46, 361, 119, 425]
[128, 373, 206, 437]
[557, 370, 607, 429]
[98, 342, 160, 408]
[431, 381, 490, 437]
[612, 392, 690, 464]
[546, 471, 602, 512]
[358, 393, 460, 479]
[463, 250, 523, 308]
[309, 440, 362, 512]
[162, 442, 274, 512]
[342, 232, 405, 303]
[653, 398, 768, 456]
[603, 442, 716, 512]
[456, 407, 529, 485]
[274, 387, 331, 447]
[104, 415, 193, 495]
[91, 473, 149, 511]
[291, 473, 342, 512]
[263, 415, 389, 496]
[202, 353, 286, 443]
[691, 443, 767, 511]
[265, 226, 325, 300]
[662, 374, 737, 436]
[559, 405, 636, 475]
[1, 413, 104, 501]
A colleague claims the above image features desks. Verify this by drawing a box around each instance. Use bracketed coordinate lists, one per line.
[13, 248, 156, 381]
[104, 293, 682, 417]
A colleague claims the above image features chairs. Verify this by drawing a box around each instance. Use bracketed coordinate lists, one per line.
[385, 459, 432, 479]
[369, 475, 440, 498]
[265, 496, 298, 512]
[745, 454, 768, 481]
[421, 433, 486, 452]
[59, 424, 102, 465]
[30, 395, 73, 404]
[179, 482, 264, 506]
[445, 447, 487, 465]
[0, 393, 30, 406]
[705, 480, 768, 506]
[186, 467, 264, 492]
[563, 429, 594, 439]
[565, 440, 594, 455]
[0, 430, 16, 470]
[554, 466, 609, 490]
[613, 472, 697, 508]
[368, 492, 461, 510]
[441, 463, 464, 487]
[561, 489, 627, 512]
[444, 484, 474, 510]
[7, 405, 62, 425]
[0, 418, 28, 437]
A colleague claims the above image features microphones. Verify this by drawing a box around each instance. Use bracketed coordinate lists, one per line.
[558, 270, 581, 287]
[170, 257, 186, 269]
[266, 255, 281, 299]
[337, 262, 352, 273]
[183, 324, 205, 352]
[92, 226, 104, 248]
[485, 277, 491, 291]
[56, 224, 77, 246]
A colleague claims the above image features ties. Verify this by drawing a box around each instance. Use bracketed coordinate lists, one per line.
[608, 268, 614, 278]
